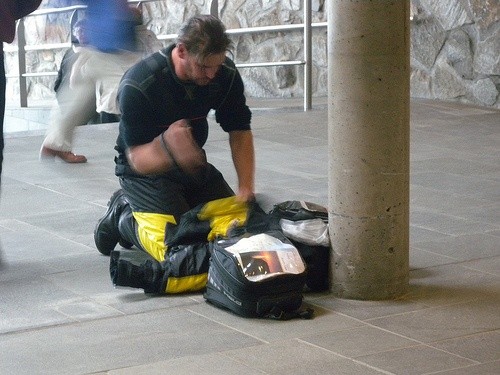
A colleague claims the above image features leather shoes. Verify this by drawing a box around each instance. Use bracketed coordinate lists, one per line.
[118, 236, 134, 249]
[93, 186, 130, 257]
[40, 145, 88, 162]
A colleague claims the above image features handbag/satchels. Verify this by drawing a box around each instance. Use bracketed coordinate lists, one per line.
[268, 198, 330, 293]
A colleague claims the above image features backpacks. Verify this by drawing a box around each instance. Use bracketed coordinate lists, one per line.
[205, 229, 315, 320]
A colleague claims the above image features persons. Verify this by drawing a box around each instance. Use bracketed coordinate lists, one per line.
[0, 0, 40, 177]
[41, 0, 149, 162]
[53, 19, 117, 124]
[94, 14, 255, 255]
[127, 8, 160, 55]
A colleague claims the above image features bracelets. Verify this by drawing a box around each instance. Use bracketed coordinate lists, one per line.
[161, 132, 178, 167]
